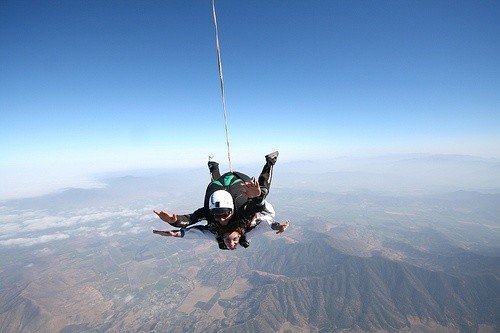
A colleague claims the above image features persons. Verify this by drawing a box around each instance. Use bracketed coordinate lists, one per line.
[152, 151, 279, 228]
[153, 200, 290, 250]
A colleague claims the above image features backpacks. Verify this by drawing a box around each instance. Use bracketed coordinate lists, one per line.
[206, 171, 256, 213]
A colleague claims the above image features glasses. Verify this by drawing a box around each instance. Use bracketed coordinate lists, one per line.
[212, 209, 232, 219]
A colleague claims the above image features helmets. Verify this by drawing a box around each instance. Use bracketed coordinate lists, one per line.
[209, 190, 234, 215]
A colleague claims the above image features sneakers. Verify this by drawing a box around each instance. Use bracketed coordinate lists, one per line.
[265, 151, 279, 164]
[208, 162, 219, 173]
